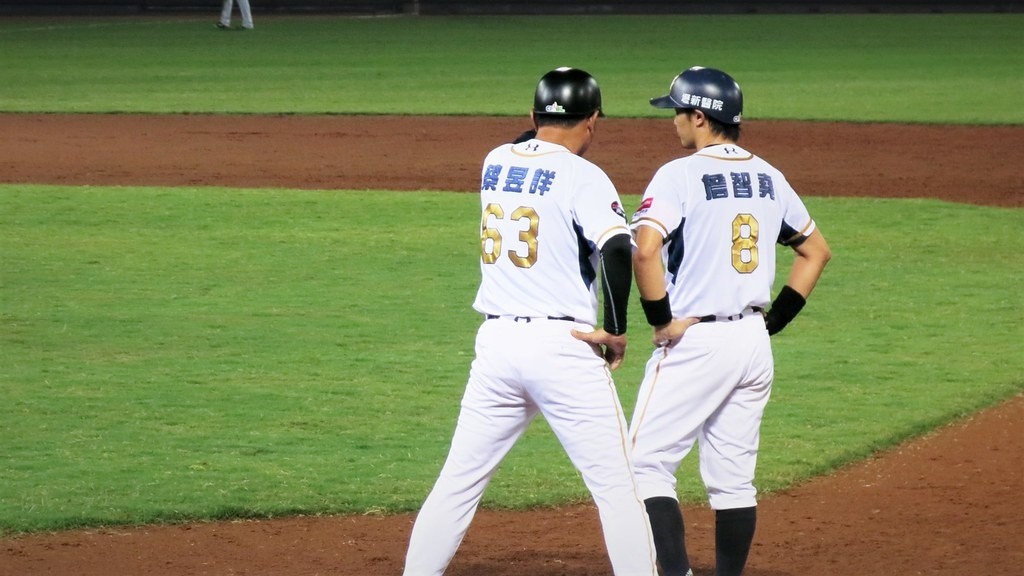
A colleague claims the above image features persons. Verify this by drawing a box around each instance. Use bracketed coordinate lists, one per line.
[211, 0, 254, 30]
[402, 66, 656, 576]
[627, 65, 834, 576]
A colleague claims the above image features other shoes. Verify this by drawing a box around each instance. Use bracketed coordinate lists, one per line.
[212, 22, 225, 28]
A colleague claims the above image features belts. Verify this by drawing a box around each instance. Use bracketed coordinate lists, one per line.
[699, 306, 761, 322]
[488, 314, 574, 322]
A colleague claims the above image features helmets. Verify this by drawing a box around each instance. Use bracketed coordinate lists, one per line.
[534, 66, 606, 119]
[650, 66, 743, 124]
